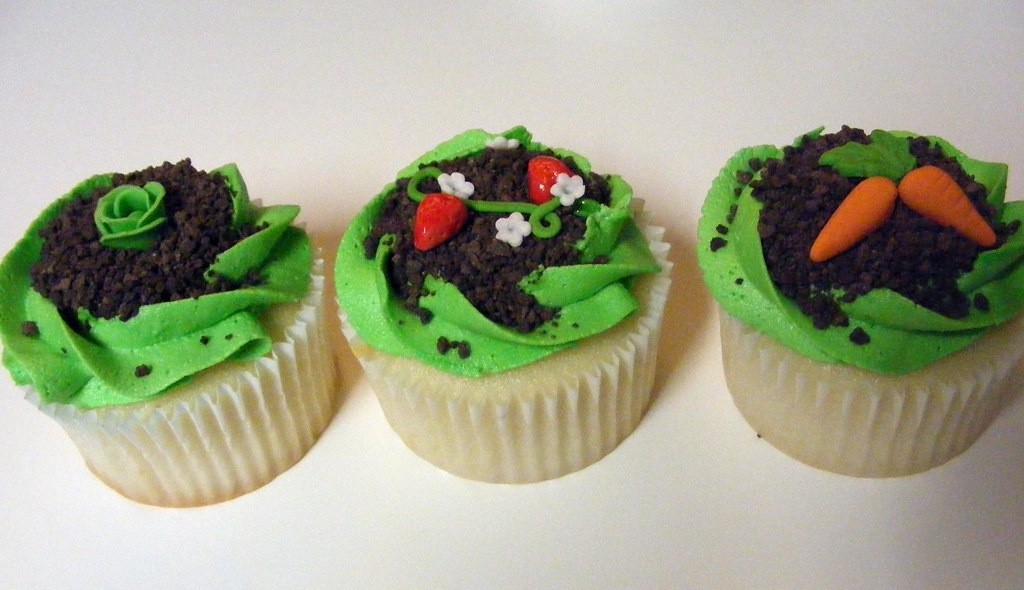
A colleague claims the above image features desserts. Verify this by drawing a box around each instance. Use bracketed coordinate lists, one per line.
[331, 125, 675, 485]
[1, 158, 339, 508]
[694, 124, 1024, 478]
